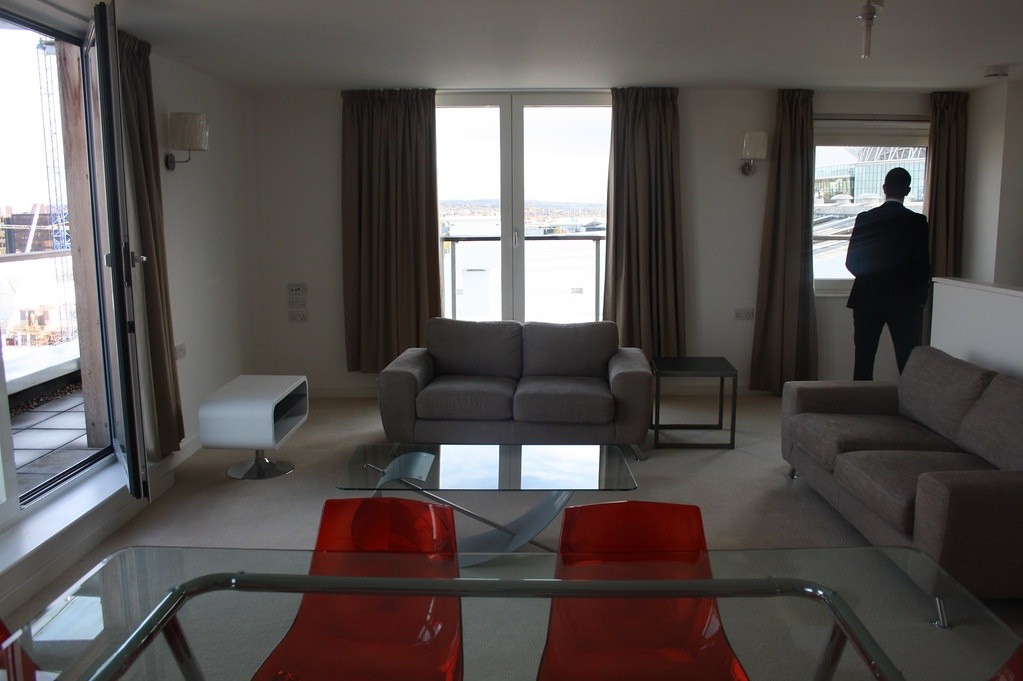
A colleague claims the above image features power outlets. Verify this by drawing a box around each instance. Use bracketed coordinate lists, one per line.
[732, 308, 754, 321]
[286, 283, 307, 295]
[287, 312, 307, 324]
[176, 342, 187, 359]
[288, 299, 306, 309]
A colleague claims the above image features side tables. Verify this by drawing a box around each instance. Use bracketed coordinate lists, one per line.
[650, 355, 737, 450]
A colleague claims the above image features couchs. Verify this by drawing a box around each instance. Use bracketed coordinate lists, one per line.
[370, 318, 654, 449]
[781, 342, 1023, 628]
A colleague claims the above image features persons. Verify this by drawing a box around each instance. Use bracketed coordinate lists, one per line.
[846, 167, 931, 380]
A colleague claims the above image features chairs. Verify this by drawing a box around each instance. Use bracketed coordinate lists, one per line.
[247, 495, 462, 681]
[533, 500, 750, 681]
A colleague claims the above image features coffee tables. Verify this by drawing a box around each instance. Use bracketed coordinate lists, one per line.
[339, 445, 637, 564]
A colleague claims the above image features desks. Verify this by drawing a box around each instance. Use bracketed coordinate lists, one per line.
[0, 546, 1023, 681]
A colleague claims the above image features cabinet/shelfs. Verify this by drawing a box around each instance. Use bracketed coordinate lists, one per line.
[197, 372, 311, 481]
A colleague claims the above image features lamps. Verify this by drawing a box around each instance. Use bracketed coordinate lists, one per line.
[165, 114, 209, 168]
[739, 131, 769, 176]
[859, 0, 880, 60]
